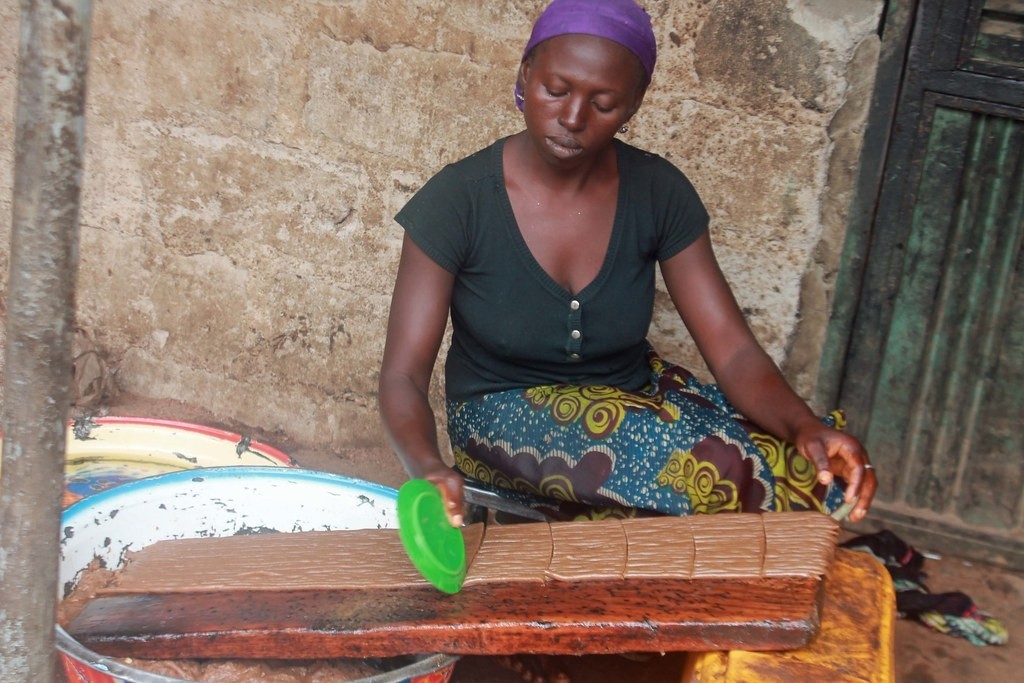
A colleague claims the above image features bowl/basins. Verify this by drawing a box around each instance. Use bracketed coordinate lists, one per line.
[57, 468, 464, 683]
[60, 419, 299, 510]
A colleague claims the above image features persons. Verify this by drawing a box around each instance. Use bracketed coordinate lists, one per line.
[379, 0, 876, 682]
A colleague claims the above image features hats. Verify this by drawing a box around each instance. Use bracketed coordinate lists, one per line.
[514, 0, 658, 114]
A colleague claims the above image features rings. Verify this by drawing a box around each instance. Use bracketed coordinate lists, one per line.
[865, 464, 875, 470]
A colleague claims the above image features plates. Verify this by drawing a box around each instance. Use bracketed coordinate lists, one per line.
[398, 477, 464, 593]
